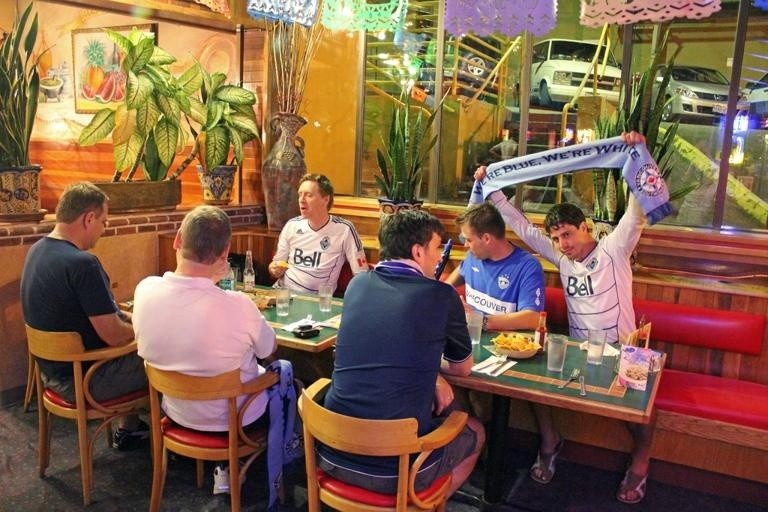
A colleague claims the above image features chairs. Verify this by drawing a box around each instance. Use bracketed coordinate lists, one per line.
[22, 321, 159, 506]
[142, 360, 304, 511]
[297, 377, 469, 511]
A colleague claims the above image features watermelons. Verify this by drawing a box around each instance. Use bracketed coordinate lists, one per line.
[82, 73, 126, 104]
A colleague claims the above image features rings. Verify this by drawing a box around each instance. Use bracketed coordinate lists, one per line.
[447, 396, 451, 400]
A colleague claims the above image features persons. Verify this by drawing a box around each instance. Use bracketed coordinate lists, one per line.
[19, 182, 149, 451]
[444, 204, 545, 331]
[268, 174, 369, 297]
[131, 205, 305, 496]
[473, 130, 658, 504]
[315, 209, 486, 503]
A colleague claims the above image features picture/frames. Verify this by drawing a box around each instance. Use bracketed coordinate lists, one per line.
[70, 21, 156, 114]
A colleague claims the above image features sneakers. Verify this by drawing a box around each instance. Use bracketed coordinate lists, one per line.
[212, 464, 247, 495]
[112, 418, 150, 452]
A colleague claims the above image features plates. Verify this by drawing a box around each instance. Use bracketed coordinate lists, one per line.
[617, 365, 646, 385]
[494, 344, 538, 359]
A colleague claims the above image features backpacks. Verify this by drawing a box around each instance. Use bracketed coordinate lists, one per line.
[227, 252, 270, 286]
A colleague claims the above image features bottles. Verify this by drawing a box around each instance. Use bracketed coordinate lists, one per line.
[218, 262, 233, 291]
[241, 251, 255, 291]
[534, 311, 547, 354]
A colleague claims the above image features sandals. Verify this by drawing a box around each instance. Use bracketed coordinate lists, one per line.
[529, 431, 564, 484]
[615, 451, 652, 503]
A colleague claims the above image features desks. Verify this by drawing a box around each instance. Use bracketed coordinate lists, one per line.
[118, 279, 345, 353]
[444, 328, 669, 505]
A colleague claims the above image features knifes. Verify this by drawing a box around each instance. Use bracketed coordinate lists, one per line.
[577, 375, 587, 396]
[490, 359, 511, 377]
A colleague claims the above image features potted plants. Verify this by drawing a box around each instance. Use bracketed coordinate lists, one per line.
[579, 20, 684, 274]
[75, 26, 208, 212]
[182, 52, 259, 206]
[0, 1, 49, 223]
[370, 82, 453, 223]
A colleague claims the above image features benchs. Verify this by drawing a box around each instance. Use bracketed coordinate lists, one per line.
[331, 261, 767, 508]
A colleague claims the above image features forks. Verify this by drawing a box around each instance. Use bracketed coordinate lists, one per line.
[474, 359, 503, 372]
[557, 368, 579, 390]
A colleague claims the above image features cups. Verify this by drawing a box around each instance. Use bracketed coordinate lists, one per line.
[230, 267, 238, 290]
[318, 283, 332, 312]
[462, 310, 483, 346]
[545, 334, 566, 372]
[275, 288, 289, 317]
[586, 328, 607, 365]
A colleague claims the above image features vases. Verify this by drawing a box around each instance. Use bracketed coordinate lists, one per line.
[261, 111, 310, 233]
